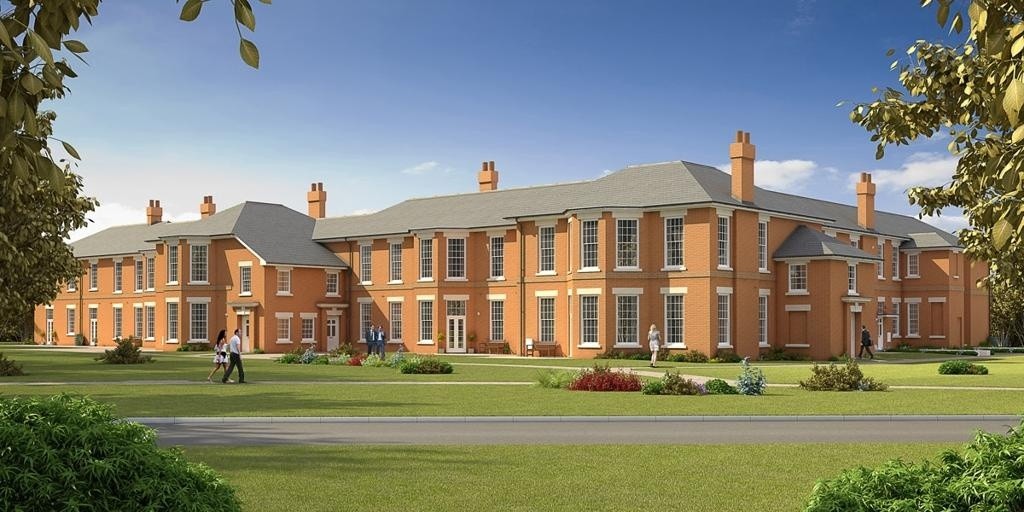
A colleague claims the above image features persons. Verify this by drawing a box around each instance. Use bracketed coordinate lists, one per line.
[222, 328, 247, 383]
[207, 330, 234, 383]
[647, 324, 661, 368]
[858, 325, 873, 360]
[365, 324, 377, 356]
[375, 324, 386, 360]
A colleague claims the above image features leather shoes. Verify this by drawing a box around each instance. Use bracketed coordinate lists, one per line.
[207, 376, 247, 384]
